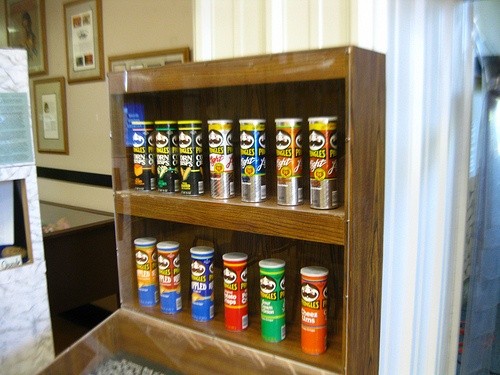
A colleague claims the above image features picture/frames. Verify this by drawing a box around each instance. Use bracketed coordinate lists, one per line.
[33, 76, 69, 156]
[64, 0, 106, 85]
[5, 0, 49, 77]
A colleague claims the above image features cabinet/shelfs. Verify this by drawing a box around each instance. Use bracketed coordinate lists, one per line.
[40, 199, 121, 313]
[108, 46, 384, 375]
